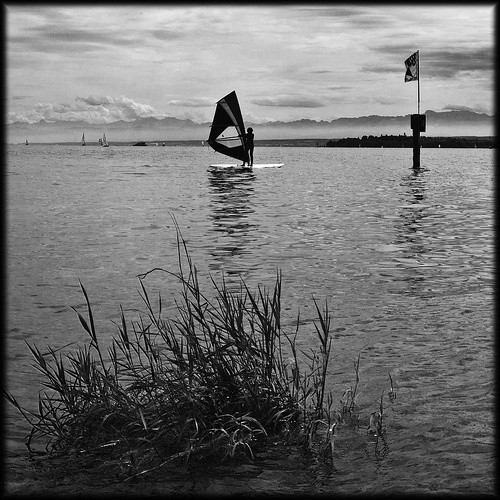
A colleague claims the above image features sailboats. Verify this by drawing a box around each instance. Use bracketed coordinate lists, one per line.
[25, 139, 29, 145]
[81, 131, 86, 146]
[205, 90, 285, 173]
[99, 133, 110, 147]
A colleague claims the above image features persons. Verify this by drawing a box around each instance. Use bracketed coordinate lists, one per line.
[237, 126, 255, 166]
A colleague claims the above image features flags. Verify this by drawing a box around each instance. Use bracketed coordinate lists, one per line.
[402, 50, 418, 82]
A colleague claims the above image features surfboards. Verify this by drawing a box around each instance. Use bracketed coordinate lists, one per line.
[209, 161, 285, 169]
